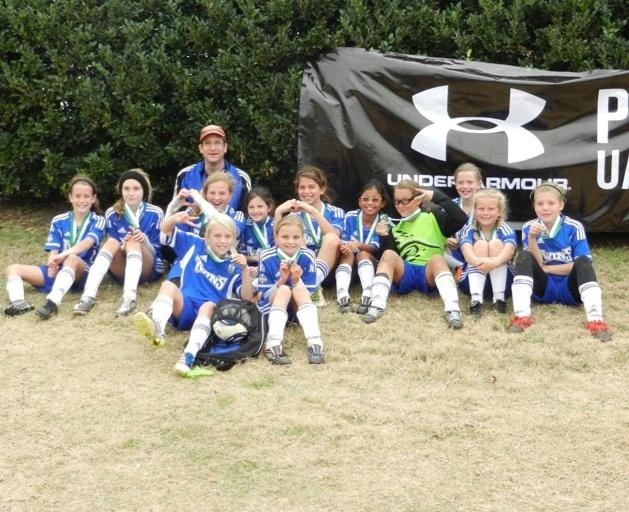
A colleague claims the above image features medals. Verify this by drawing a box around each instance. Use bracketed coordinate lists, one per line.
[226, 264, 237, 276]
[285, 259, 294, 266]
[380, 214, 388, 222]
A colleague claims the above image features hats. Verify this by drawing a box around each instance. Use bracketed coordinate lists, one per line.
[200, 125, 225, 141]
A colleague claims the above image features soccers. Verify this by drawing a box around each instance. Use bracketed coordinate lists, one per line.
[213, 304, 251, 342]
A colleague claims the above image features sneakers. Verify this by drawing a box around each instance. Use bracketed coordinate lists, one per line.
[3, 287, 612, 376]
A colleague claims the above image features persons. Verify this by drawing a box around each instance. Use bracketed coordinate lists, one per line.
[1, 173, 106, 319]
[274, 166, 345, 308]
[334, 178, 391, 314]
[257, 214, 325, 365]
[361, 179, 468, 329]
[172, 124, 252, 215]
[71, 167, 165, 316]
[133, 212, 254, 377]
[456, 188, 518, 322]
[441, 162, 484, 271]
[233, 186, 277, 277]
[506, 182, 613, 343]
[165, 171, 247, 287]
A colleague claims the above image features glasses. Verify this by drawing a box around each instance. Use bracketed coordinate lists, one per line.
[392, 194, 421, 205]
